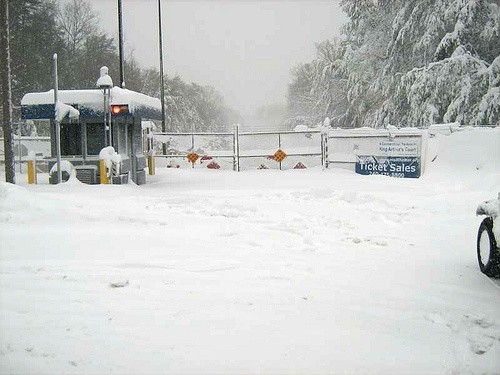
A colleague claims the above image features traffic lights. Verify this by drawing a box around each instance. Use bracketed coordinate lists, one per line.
[111, 104, 129, 115]
